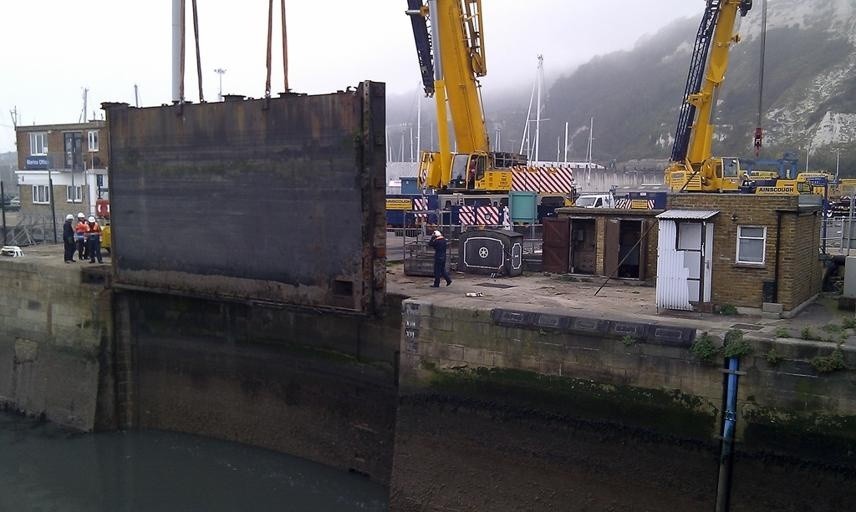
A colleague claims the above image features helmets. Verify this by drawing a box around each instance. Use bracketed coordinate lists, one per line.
[87, 216, 94, 222]
[66, 214, 73, 220]
[77, 212, 84, 218]
[432, 230, 441, 237]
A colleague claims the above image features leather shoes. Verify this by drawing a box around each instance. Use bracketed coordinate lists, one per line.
[446, 280, 451, 286]
[430, 284, 434, 287]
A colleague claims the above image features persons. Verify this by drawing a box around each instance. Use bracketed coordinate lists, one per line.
[73, 212, 89, 260]
[62, 214, 77, 264]
[428, 229, 452, 288]
[83, 216, 104, 264]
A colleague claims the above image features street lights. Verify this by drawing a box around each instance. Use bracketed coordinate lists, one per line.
[213, 68, 224, 101]
[801, 145, 813, 173]
[829, 146, 846, 176]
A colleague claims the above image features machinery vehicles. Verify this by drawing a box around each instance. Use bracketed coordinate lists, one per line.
[403, 1, 575, 230]
[664, 0, 814, 196]
[95, 187, 111, 254]
[741, 170, 855, 200]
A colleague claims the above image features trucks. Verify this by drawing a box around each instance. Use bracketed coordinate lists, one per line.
[570, 191, 615, 210]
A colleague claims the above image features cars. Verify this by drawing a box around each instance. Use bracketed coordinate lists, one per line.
[821, 201, 856, 218]
[4, 196, 20, 211]
